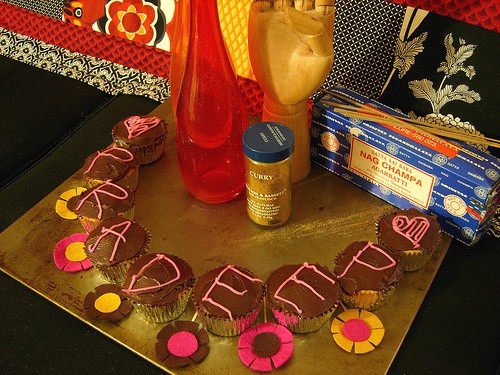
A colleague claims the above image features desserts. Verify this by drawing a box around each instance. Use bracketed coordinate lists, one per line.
[71, 116, 442, 338]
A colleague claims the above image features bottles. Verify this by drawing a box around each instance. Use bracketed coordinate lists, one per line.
[175, 0, 249, 205]
[241, 122, 296, 227]
